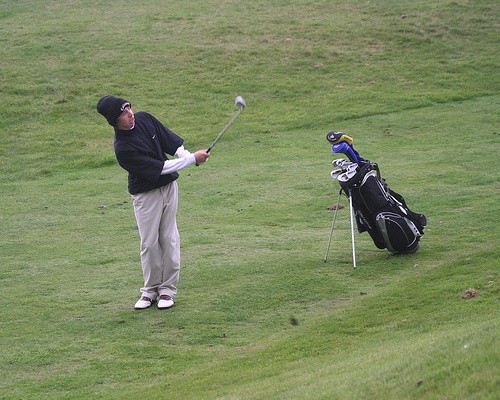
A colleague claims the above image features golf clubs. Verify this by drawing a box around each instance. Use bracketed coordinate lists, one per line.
[325, 130, 363, 185]
[194, 95, 248, 169]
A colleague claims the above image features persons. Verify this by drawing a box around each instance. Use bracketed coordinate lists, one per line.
[97, 94, 210, 309]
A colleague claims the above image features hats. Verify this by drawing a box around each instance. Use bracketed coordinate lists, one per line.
[97, 95, 131, 126]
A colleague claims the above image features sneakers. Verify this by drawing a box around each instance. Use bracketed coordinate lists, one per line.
[158, 295, 174, 310]
[135, 296, 152, 309]
